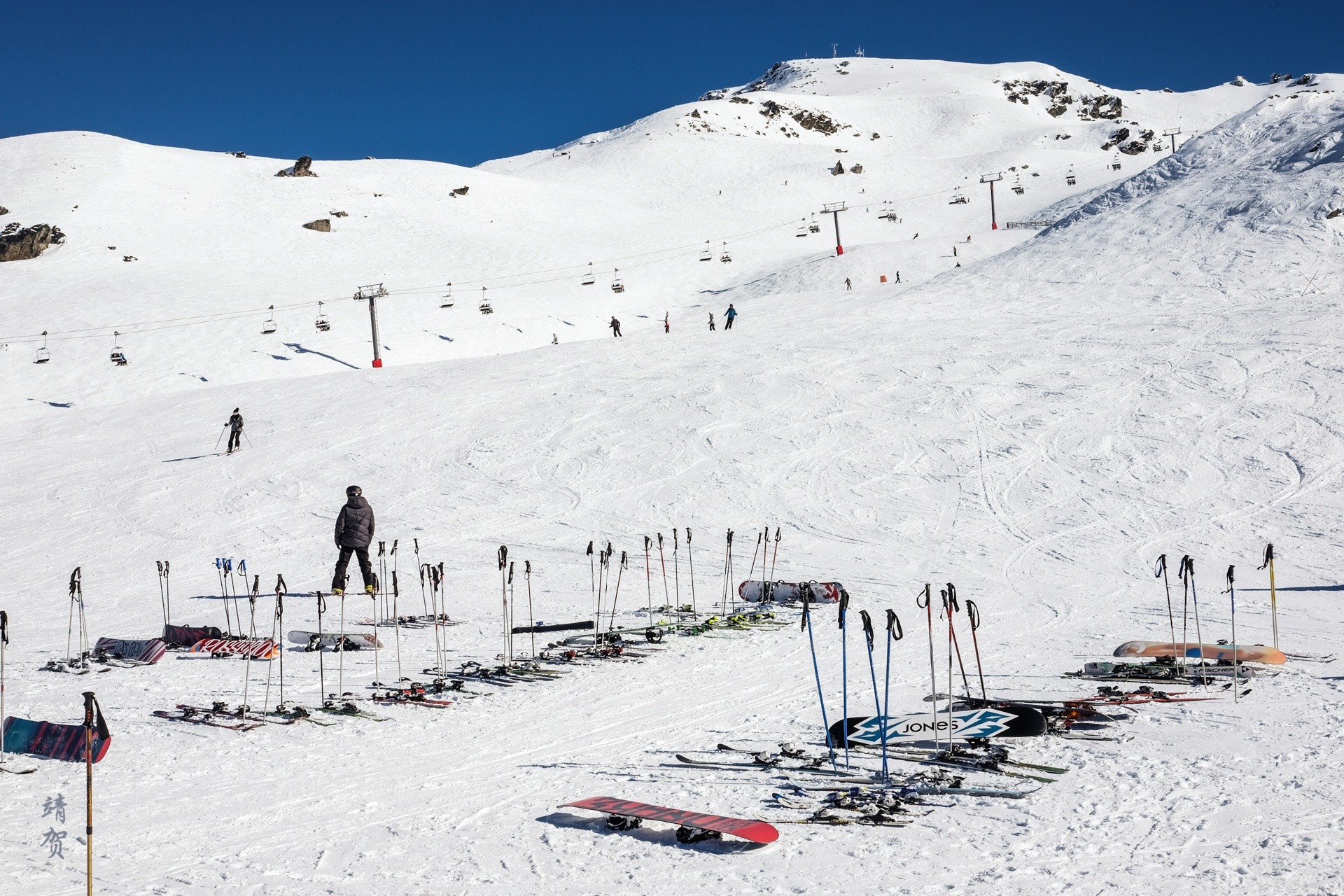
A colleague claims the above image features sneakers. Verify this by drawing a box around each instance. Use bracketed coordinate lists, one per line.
[365, 585, 373, 595]
[332, 588, 343, 596]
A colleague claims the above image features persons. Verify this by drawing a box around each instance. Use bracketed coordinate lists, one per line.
[887, 213, 902, 223]
[331, 481, 375, 595]
[912, 233, 918, 239]
[609, 316, 622, 337]
[867, 207, 869, 213]
[1015, 187, 1024, 195]
[955, 262, 960, 268]
[707, 303, 737, 331]
[225, 407, 245, 453]
[722, 255, 732, 262]
[111, 352, 128, 366]
[316, 320, 330, 329]
[953, 246, 957, 256]
[966, 235, 971, 242]
[955, 197, 970, 204]
[613, 283, 621, 293]
[552, 333, 558, 344]
[862, 188, 864, 193]
[664, 311, 670, 334]
[844, 277, 852, 290]
[785, 180, 787, 185]
[1068, 179, 1076, 185]
[810, 225, 820, 233]
[896, 271, 900, 283]
[481, 304, 489, 314]
[719, 190, 722, 195]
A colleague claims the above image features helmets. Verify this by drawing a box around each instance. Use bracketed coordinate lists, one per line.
[347, 485, 362, 496]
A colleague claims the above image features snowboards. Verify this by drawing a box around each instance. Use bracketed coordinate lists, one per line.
[1114, 640, 1286, 664]
[91, 637, 167, 663]
[162, 625, 223, 646]
[288, 631, 384, 652]
[739, 580, 843, 604]
[923, 693, 1138, 731]
[825, 706, 1048, 749]
[0, 717, 110, 763]
[190, 638, 285, 660]
[557, 797, 779, 844]
[511, 621, 597, 634]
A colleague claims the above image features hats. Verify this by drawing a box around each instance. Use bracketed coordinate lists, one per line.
[233, 408, 239, 413]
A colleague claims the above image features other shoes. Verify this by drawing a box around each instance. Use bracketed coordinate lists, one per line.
[234, 446, 239, 450]
[226, 448, 231, 453]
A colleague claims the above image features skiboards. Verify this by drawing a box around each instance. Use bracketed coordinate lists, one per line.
[359, 614, 463, 629]
[153, 699, 296, 732]
[658, 610, 804, 632]
[1073, 686, 1225, 706]
[277, 659, 573, 724]
[675, 743, 1069, 797]
[758, 787, 956, 828]
[539, 640, 668, 666]
[217, 449, 243, 455]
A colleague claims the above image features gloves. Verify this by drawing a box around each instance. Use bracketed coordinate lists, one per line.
[337, 545, 341, 550]
[238, 425, 241, 428]
[225, 423, 228, 426]
